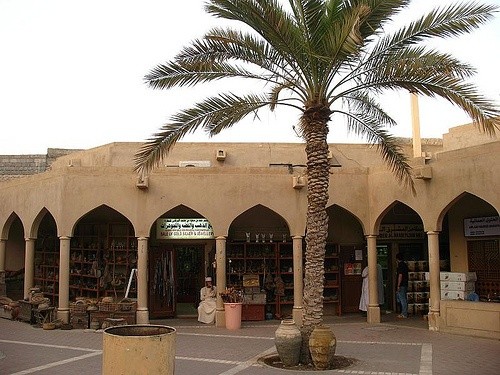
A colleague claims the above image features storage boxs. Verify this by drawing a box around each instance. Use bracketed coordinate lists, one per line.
[440, 272, 477, 300]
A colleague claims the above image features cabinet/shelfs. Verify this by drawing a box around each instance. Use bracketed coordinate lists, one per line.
[105, 222, 137, 297]
[226, 242, 277, 320]
[70, 222, 105, 298]
[278, 242, 341, 316]
[34, 237, 60, 306]
[407, 260, 429, 305]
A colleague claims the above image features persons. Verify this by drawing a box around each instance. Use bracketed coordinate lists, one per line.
[197, 277, 216, 324]
[395, 253, 409, 319]
[358, 264, 384, 316]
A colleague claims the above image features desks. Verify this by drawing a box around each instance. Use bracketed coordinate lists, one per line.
[32, 307, 53, 326]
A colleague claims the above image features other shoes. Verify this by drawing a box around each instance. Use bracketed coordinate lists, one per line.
[396, 313, 407, 318]
